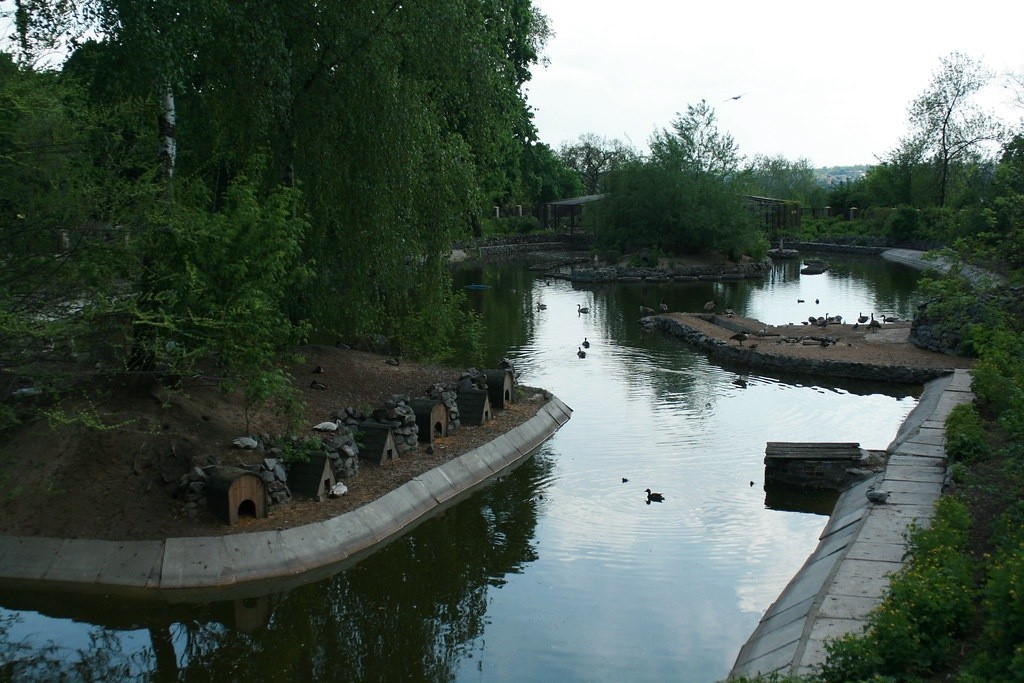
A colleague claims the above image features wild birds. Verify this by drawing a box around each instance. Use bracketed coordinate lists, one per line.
[723, 307, 737, 319]
[535, 307, 547, 312]
[702, 300, 716, 313]
[796, 298, 806, 304]
[576, 303, 589, 311]
[807, 310, 900, 334]
[309, 364, 329, 392]
[233, 434, 259, 449]
[639, 298, 668, 317]
[534, 300, 548, 307]
[728, 330, 750, 346]
[864, 482, 894, 503]
[576, 347, 586, 355]
[815, 298, 820, 304]
[731, 373, 748, 387]
[643, 489, 664, 497]
[772, 333, 842, 349]
[621, 477, 629, 482]
[328, 482, 348, 497]
[578, 311, 588, 318]
[312, 419, 342, 433]
[583, 338, 590, 346]
[383, 355, 403, 366]
